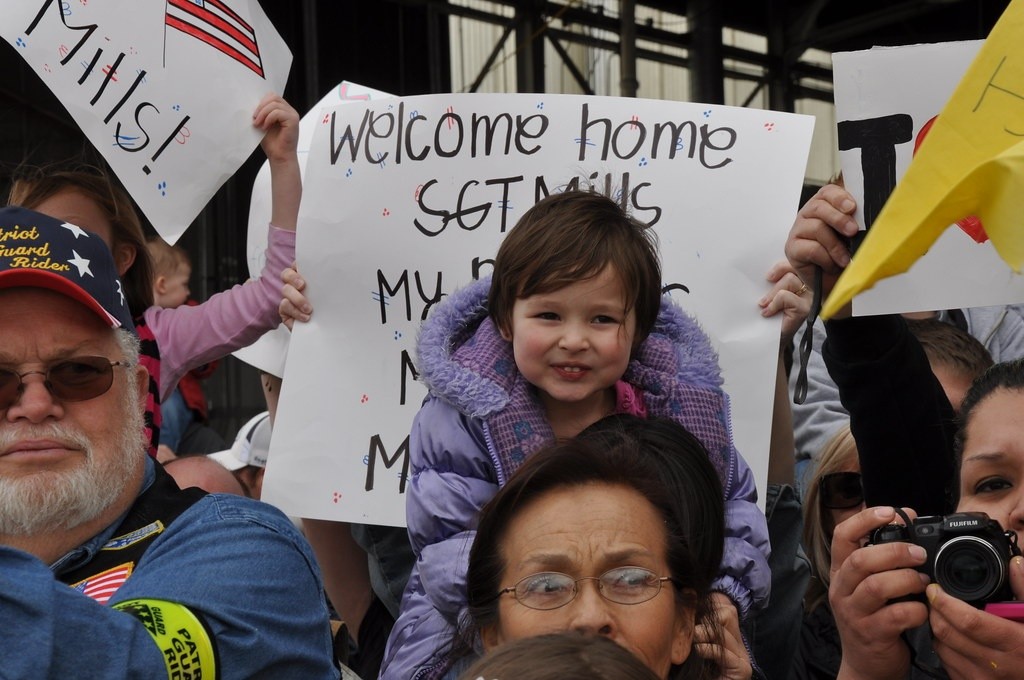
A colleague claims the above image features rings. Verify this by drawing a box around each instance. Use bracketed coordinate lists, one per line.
[797, 284, 807, 296]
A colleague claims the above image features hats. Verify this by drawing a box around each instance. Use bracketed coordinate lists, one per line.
[1, 205, 139, 330]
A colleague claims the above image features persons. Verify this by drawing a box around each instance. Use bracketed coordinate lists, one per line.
[783, 170, 1023, 679]
[0, 206, 341, 680]
[138, 235, 192, 309]
[802, 422, 903, 680]
[1, 91, 302, 498]
[377, 188, 772, 680]
[278, 245, 816, 680]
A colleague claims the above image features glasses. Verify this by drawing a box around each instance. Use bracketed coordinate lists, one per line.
[496, 564, 675, 611]
[0, 356, 114, 412]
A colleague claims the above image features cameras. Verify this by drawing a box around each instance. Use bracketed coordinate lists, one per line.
[863, 512, 1014, 611]
